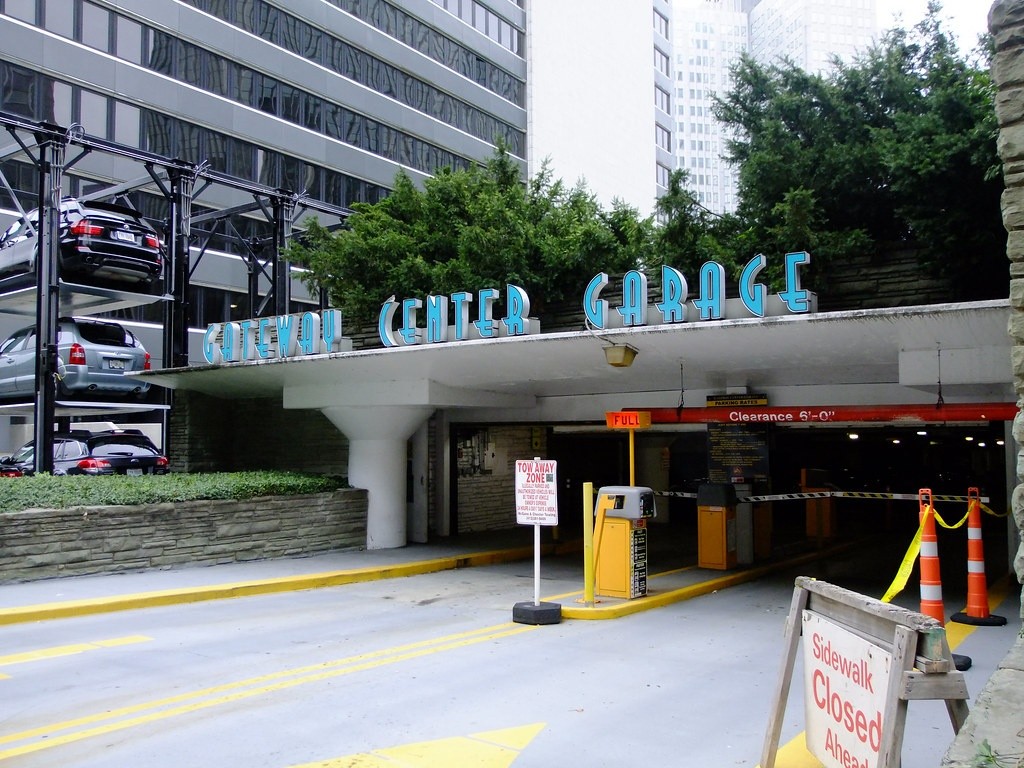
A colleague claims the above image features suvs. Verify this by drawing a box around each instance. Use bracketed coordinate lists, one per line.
[1, 196, 163, 292]
[0, 428, 167, 478]
[2, 313, 153, 397]
